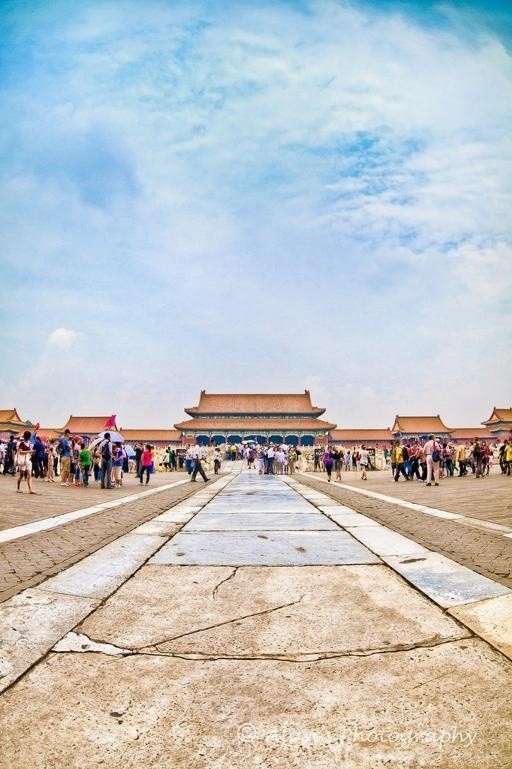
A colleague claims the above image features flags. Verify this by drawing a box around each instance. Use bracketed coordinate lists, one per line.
[104, 416, 116, 428]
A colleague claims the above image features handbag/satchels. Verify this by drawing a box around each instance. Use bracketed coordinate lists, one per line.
[14, 453, 32, 466]
[432, 452, 441, 462]
[102, 445, 110, 459]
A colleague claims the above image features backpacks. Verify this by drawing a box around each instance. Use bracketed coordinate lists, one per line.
[399, 446, 409, 460]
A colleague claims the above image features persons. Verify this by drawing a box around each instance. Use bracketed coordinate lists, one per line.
[0, 434, 59, 483]
[226, 440, 302, 476]
[16, 431, 37, 494]
[383, 433, 511, 487]
[53, 428, 155, 490]
[312, 444, 375, 483]
[162, 444, 223, 482]
